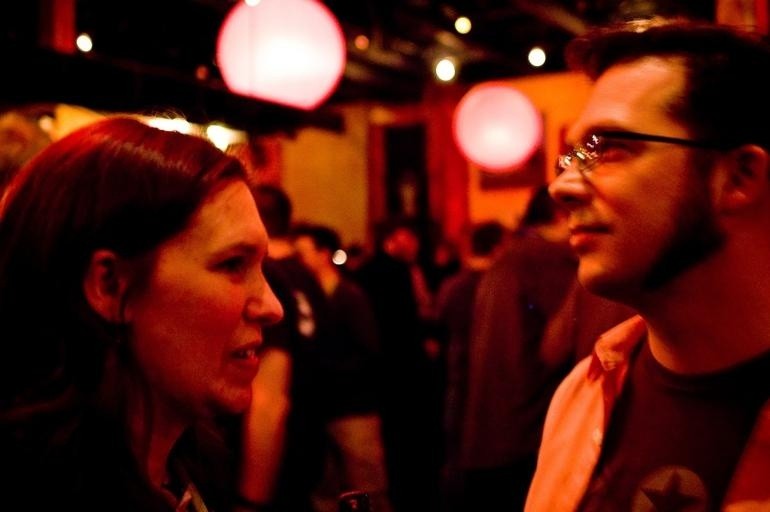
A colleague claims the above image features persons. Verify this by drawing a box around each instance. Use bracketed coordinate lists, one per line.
[519, 20, 769, 510]
[232, 166, 608, 512]
[1, 114, 288, 508]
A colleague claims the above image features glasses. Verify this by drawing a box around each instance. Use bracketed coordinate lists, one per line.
[555, 128, 696, 174]
